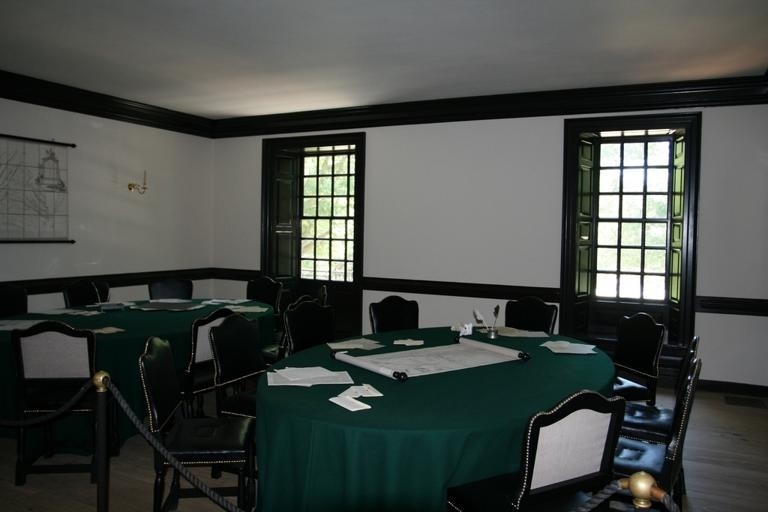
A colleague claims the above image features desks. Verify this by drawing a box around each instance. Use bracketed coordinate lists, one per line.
[257, 327, 614, 512]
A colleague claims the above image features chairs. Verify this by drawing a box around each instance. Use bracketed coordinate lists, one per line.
[139, 336, 257, 512]
[6, 320, 121, 485]
[594, 313, 665, 406]
[610, 357, 701, 512]
[1, 271, 338, 478]
[369, 296, 419, 333]
[505, 296, 558, 335]
[444, 389, 626, 512]
[620, 337, 700, 496]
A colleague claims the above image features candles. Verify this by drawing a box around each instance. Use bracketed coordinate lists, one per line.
[143, 167, 146, 186]
